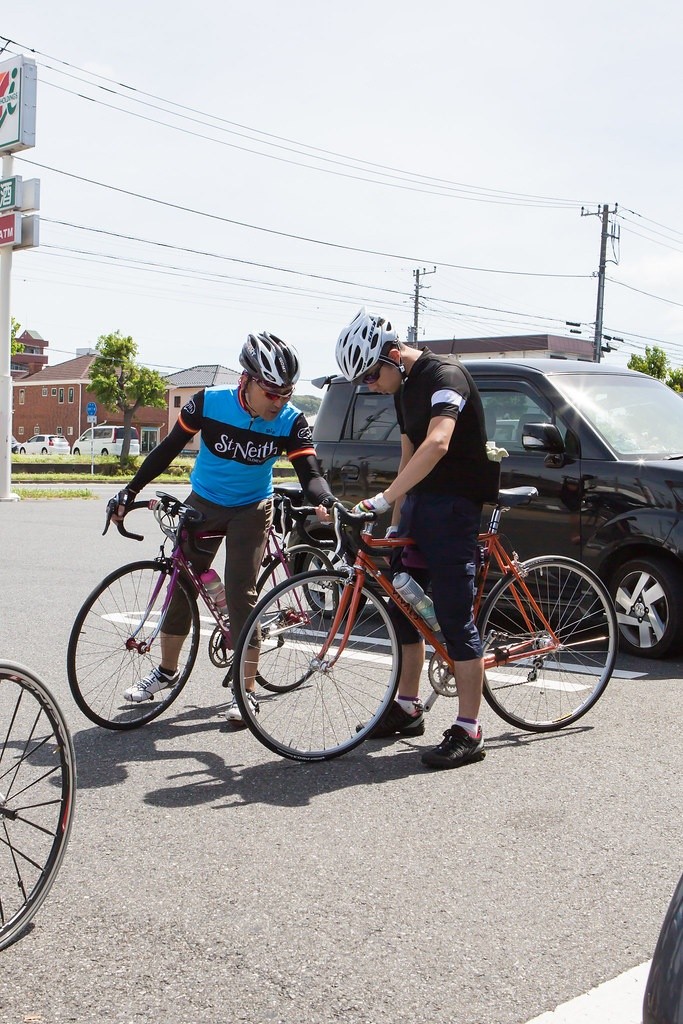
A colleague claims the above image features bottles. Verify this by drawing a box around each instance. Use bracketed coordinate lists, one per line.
[391, 572, 442, 633]
[199, 570, 229, 615]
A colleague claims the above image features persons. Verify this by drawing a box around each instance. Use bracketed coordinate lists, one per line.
[335, 306, 491, 767]
[106, 331, 343, 721]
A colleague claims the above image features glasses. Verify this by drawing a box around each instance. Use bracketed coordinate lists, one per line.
[252, 376, 294, 402]
[362, 347, 391, 384]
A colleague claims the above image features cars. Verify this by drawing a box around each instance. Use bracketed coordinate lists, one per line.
[11, 436, 21, 454]
[19, 435, 71, 455]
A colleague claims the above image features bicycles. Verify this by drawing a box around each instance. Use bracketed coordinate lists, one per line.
[66, 490, 341, 731]
[0, 660, 78, 953]
[232, 486, 620, 761]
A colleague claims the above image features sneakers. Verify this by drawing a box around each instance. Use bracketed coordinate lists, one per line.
[421, 727, 485, 769]
[354, 699, 426, 737]
[122, 666, 180, 701]
[225, 690, 259, 720]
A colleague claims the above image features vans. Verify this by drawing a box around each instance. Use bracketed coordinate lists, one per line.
[72, 426, 141, 458]
[299, 359, 683, 659]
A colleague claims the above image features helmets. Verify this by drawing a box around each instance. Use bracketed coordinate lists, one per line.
[238, 330, 299, 387]
[335, 307, 396, 381]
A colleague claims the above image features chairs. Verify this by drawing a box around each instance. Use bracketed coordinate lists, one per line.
[484, 408, 496, 441]
[515, 414, 550, 441]
